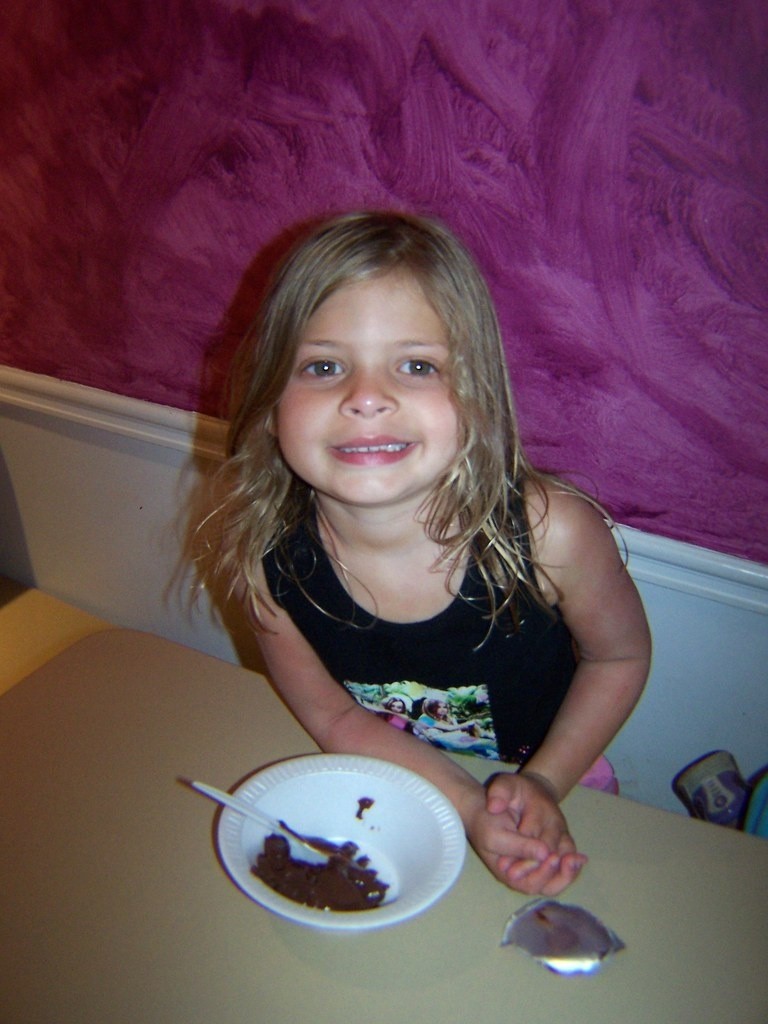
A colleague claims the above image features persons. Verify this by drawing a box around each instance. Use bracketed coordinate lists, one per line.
[186, 211, 652, 898]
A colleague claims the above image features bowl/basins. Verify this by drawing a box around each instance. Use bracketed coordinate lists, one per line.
[218, 753, 466, 931]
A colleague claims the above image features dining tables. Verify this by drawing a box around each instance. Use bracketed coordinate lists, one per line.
[0, 569, 768, 1024]
